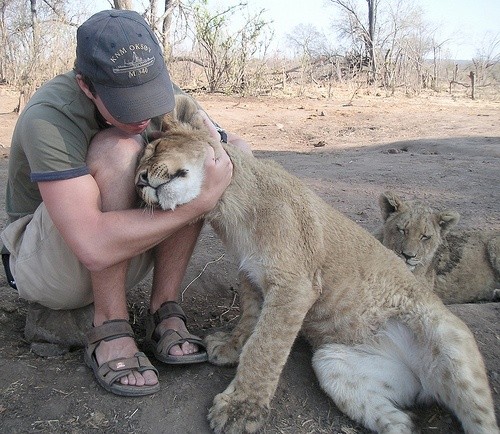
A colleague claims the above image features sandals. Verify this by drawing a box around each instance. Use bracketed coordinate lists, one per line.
[142, 301, 209, 364]
[83, 320, 161, 397]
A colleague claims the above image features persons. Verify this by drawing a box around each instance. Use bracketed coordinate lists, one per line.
[0, 8, 254, 399]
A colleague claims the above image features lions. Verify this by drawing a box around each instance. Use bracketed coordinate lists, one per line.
[134, 94, 500, 433]
[371, 192, 500, 304]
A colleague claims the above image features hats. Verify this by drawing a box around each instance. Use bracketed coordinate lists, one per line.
[76, 10, 175, 124]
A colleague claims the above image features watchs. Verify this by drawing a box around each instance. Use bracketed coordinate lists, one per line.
[215, 126, 227, 144]
